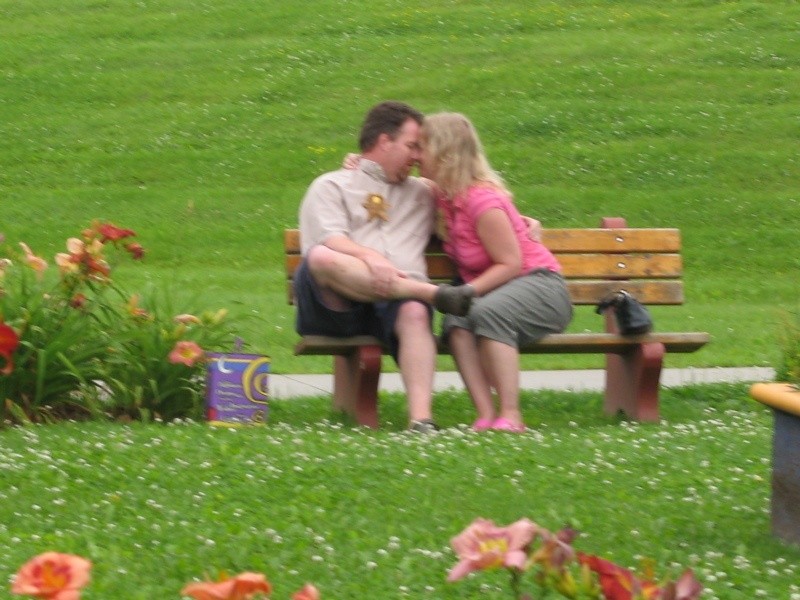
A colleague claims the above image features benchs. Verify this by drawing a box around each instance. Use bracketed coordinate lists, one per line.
[282, 214, 714, 423]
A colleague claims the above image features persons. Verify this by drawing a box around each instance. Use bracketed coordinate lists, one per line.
[294, 101, 476, 436]
[342, 112, 573, 434]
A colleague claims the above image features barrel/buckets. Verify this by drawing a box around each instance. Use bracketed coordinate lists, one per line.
[206, 352, 271, 429]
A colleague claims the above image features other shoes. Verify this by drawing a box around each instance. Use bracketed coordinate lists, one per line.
[414, 420, 439, 436]
[472, 420, 492, 429]
[433, 282, 474, 316]
[493, 418, 525, 433]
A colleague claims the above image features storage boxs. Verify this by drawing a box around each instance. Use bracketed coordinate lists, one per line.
[207, 354, 271, 430]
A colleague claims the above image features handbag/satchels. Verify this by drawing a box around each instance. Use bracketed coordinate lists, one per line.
[596, 289, 654, 335]
